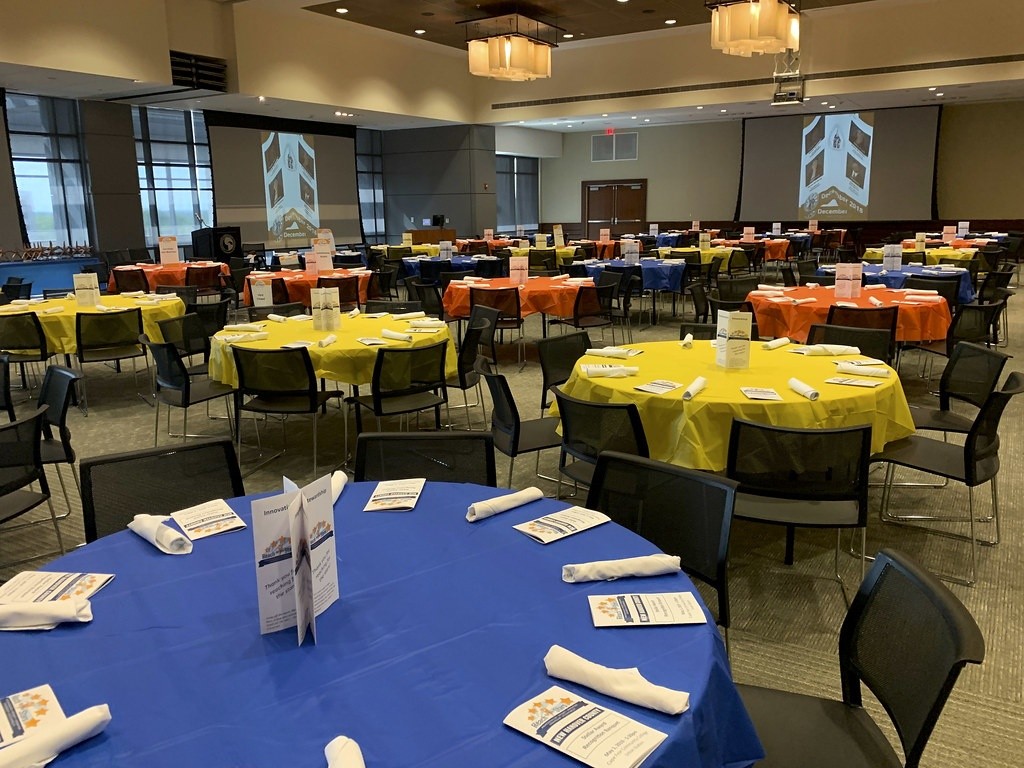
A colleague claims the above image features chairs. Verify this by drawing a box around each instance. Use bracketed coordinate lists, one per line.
[0, 232, 1024, 610]
[721, 550, 987, 768]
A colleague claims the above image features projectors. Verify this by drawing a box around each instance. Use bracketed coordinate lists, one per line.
[774, 91, 803, 103]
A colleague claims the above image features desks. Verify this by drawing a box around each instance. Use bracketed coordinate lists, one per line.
[403, 255, 504, 280]
[465, 238, 513, 251]
[659, 246, 744, 272]
[574, 257, 686, 295]
[901, 238, 995, 252]
[1, 482, 764, 768]
[301, 251, 360, 263]
[817, 262, 974, 308]
[563, 340, 916, 474]
[712, 239, 789, 262]
[243, 268, 373, 308]
[443, 277, 601, 320]
[568, 240, 627, 257]
[507, 246, 577, 269]
[745, 281, 952, 353]
[0, 294, 187, 358]
[104, 262, 230, 289]
[392, 246, 441, 256]
[209, 311, 459, 392]
[761, 233, 814, 250]
[864, 247, 979, 267]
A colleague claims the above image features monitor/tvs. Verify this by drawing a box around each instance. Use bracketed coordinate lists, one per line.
[432, 215, 444, 229]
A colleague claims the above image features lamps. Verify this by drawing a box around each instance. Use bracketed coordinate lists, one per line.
[456, 11, 567, 81]
[704, 0, 801, 58]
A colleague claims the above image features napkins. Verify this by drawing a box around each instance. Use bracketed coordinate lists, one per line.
[133, 301, 159, 306]
[146, 293, 176, 300]
[95, 305, 109, 311]
[66, 293, 75, 299]
[120, 291, 143, 298]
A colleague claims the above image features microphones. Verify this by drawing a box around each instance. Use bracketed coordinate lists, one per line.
[194, 212, 201, 223]
